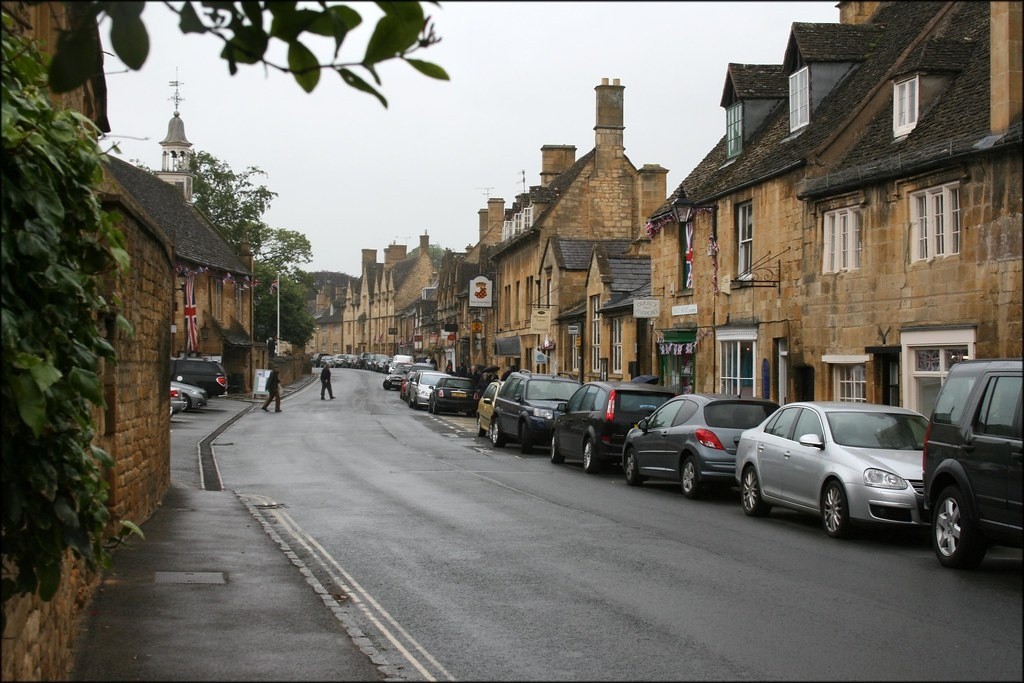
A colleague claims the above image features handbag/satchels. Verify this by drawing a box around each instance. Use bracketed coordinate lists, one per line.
[473, 392, 479, 399]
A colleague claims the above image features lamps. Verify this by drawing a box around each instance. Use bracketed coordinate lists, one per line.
[173, 266, 189, 293]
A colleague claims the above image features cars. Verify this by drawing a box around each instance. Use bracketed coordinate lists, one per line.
[170, 387, 187, 414]
[476, 378, 508, 442]
[428, 377, 479, 419]
[310, 352, 452, 410]
[736, 401, 935, 541]
[549, 380, 679, 476]
[621, 393, 782, 501]
[170, 381, 208, 413]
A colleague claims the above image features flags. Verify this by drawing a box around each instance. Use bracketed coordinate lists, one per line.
[183, 273, 199, 354]
[271, 277, 278, 292]
[657, 336, 692, 355]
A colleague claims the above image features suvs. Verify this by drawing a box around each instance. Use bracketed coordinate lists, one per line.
[170, 358, 228, 398]
[491, 368, 585, 455]
[922, 357, 1024, 573]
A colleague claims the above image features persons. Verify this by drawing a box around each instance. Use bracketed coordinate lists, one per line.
[262, 365, 282, 412]
[446, 359, 516, 399]
[416, 355, 437, 370]
[321, 364, 336, 400]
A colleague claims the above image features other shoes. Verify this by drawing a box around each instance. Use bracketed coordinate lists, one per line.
[321, 397, 325, 400]
[261, 407, 269, 411]
[275, 409, 282, 412]
[330, 397, 336, 399]
